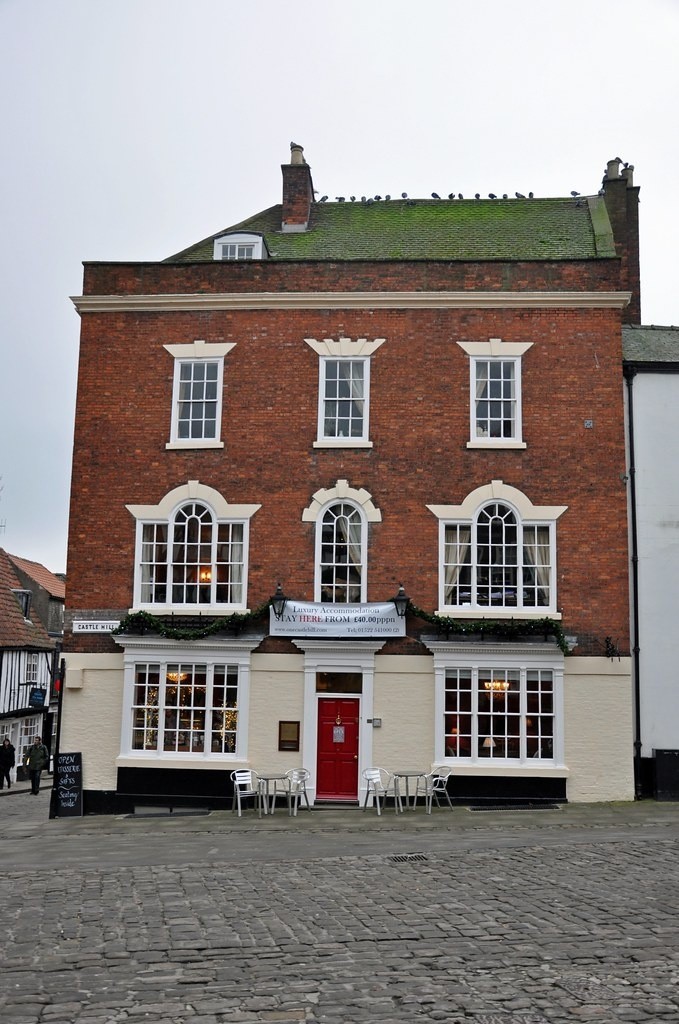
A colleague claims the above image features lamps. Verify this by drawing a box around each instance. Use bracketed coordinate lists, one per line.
[269, 583, 287, 621]
[167, 673, 187, 683]
[483, 738, 496, 747]
[484, 680, 510, 698]
[393, 583, 412, 620]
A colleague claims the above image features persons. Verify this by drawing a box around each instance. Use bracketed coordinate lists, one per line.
[448, 728, 471, 757]
[0, 739, 15, 789]
[22, 736, 49, 796]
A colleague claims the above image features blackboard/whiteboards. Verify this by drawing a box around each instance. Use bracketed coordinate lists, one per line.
[53, 752, 83, 817]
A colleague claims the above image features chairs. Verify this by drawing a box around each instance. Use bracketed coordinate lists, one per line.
[230, 769, 268, 818]
[362, 767, 404, 816]
[271, 767, 311, 817]
[412, 766, 454, 815]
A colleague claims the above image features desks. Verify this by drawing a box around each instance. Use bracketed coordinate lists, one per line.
[393, 770, 429, 816]
[256, 773, 292, 819]
[507, 599, 543, 606]
[452, 597, 498, 605]
